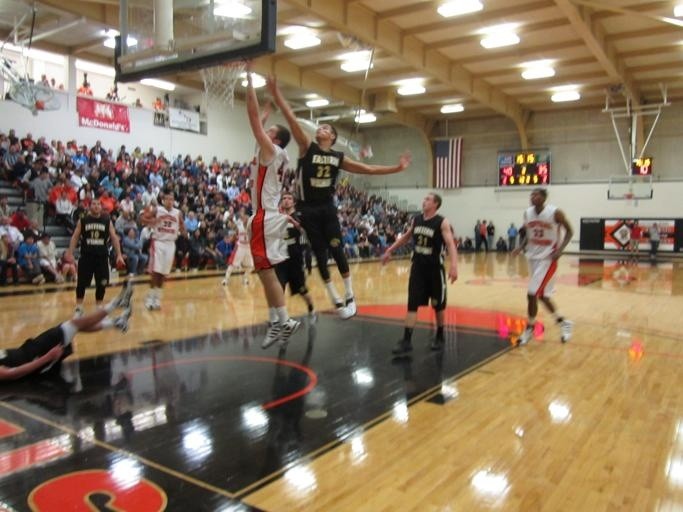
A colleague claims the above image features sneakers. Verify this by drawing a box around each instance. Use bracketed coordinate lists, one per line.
[344, 294, 357, 317]
[117, 278, 134, 310]
[308, 308, 317, 325]
[515, 326, 536, 346]
[390, 340, 414, 354]
[558, 319, 574, 342]
[430, 335, 446, 350]
[333, 296, 350, 320]
[145, 295, 162, 311]
[260, 320, 281, 350]
[220, 276, 250, 286]
[113, 305, 132, 334]
[277, 318, 302, 345]
[71, 306, 85, 319]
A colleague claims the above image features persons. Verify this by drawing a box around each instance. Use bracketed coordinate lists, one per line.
[649, 223, 661, 258]
[513, 189, 575, 343]
[629, 220, 641, 256]
